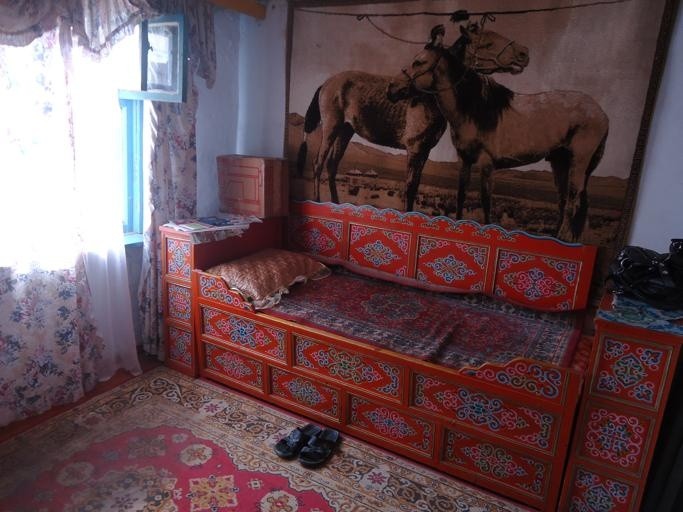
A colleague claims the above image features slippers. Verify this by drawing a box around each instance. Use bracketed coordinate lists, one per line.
[274, 424, 321, 457]
[299, 428, 339, 467]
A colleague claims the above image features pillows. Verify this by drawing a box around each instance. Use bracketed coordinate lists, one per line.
[204, 247, 331, 310]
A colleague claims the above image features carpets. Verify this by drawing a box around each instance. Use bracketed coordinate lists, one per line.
[0, 365, 542, 512]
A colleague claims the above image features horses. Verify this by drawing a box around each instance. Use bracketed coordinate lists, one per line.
[385, 22, 611, 245]
[294, 14, 530, 214]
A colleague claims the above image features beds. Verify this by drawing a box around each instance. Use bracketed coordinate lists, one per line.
[158, 197, 683, 512]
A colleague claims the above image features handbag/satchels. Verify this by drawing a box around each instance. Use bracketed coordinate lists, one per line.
[604, 247, 682, 310]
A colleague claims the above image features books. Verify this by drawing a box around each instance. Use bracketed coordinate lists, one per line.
[161, 212, 263, 234]
